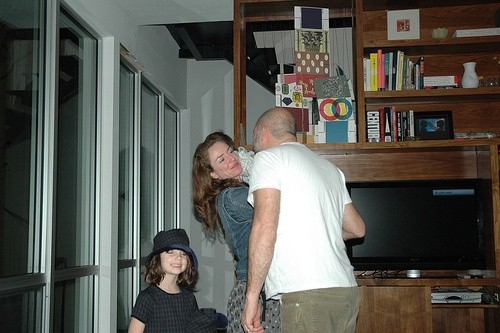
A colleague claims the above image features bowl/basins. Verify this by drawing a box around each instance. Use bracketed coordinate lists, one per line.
[431, 27, 448, 38]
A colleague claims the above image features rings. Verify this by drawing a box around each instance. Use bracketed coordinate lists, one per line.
[246, 329, 250, 333]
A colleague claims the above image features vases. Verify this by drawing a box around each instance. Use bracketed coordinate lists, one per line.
[461, 62, 479, 88]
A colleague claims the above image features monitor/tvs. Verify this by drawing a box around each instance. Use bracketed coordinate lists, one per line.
[345, 178, 495, 271]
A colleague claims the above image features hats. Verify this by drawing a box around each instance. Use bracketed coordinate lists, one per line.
[145, 229, 198, 271]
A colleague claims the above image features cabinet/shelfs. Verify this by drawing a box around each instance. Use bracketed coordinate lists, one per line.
[234, 0, 500, 333]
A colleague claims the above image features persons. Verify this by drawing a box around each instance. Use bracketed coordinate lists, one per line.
[128, 229, 199, 333]
[240, 107, 366, 333]
[190, 132, 281, 333]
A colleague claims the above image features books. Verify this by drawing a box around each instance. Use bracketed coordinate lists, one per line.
[379, 105, 414, 143]
[363, 49, 425, 91]
